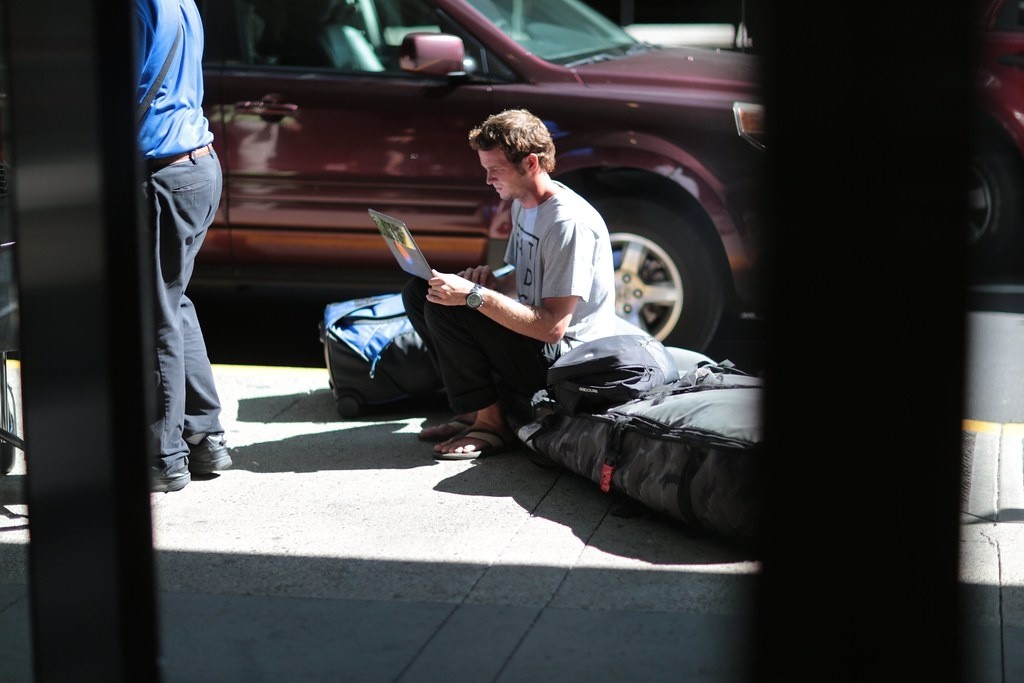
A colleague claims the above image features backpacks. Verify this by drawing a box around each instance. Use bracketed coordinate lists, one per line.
[546, 331, 681, 415]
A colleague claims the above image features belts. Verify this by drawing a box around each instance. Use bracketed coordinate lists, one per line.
[147, 144, 217, 172]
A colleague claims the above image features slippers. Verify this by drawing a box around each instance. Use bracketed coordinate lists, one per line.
[417, 419, 474, 442]
[432, 427, 513, 461]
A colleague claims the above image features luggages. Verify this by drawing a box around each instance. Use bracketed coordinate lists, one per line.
[318, 294, 438, 421]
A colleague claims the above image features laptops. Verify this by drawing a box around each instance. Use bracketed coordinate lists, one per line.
[368, 209, 435, 281]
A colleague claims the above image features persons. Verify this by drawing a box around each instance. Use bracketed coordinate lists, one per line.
[402, 109, 618, 460]
[129, 1, 234, 493]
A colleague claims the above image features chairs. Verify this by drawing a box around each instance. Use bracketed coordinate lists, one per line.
[322, 24, 385, 72]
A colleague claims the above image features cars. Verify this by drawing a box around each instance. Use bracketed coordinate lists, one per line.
[0, 0, 772, 355]
[961, 1, 1022, 278]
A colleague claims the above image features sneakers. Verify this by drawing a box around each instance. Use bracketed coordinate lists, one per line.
[144, 463, 185, 496]
[186, 432, 235, 475]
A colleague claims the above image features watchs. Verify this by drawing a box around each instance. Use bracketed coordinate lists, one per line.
[465, 283, 484, 310]
